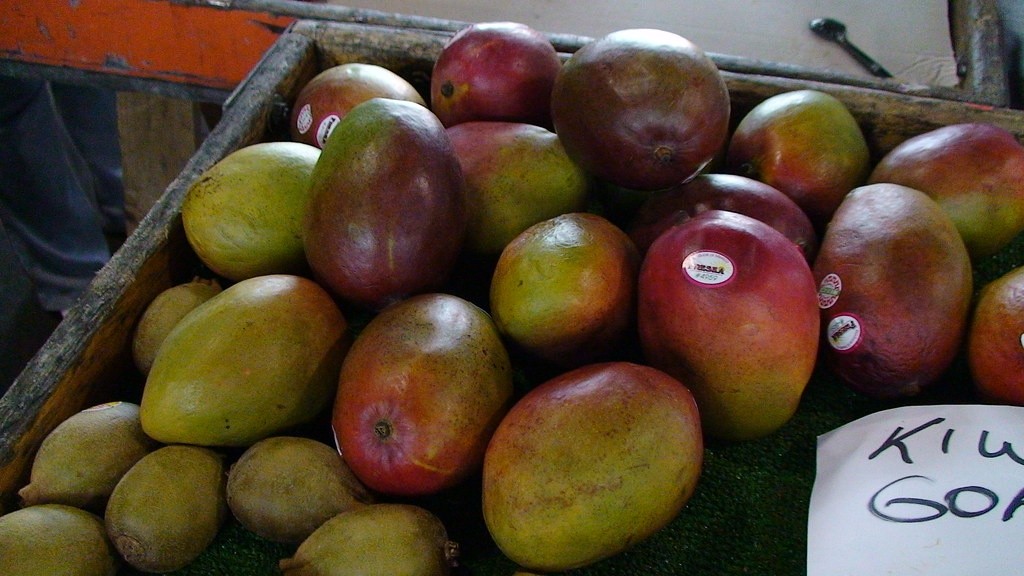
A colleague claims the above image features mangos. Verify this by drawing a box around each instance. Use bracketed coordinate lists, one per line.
[0, 21, 1024, 576]
